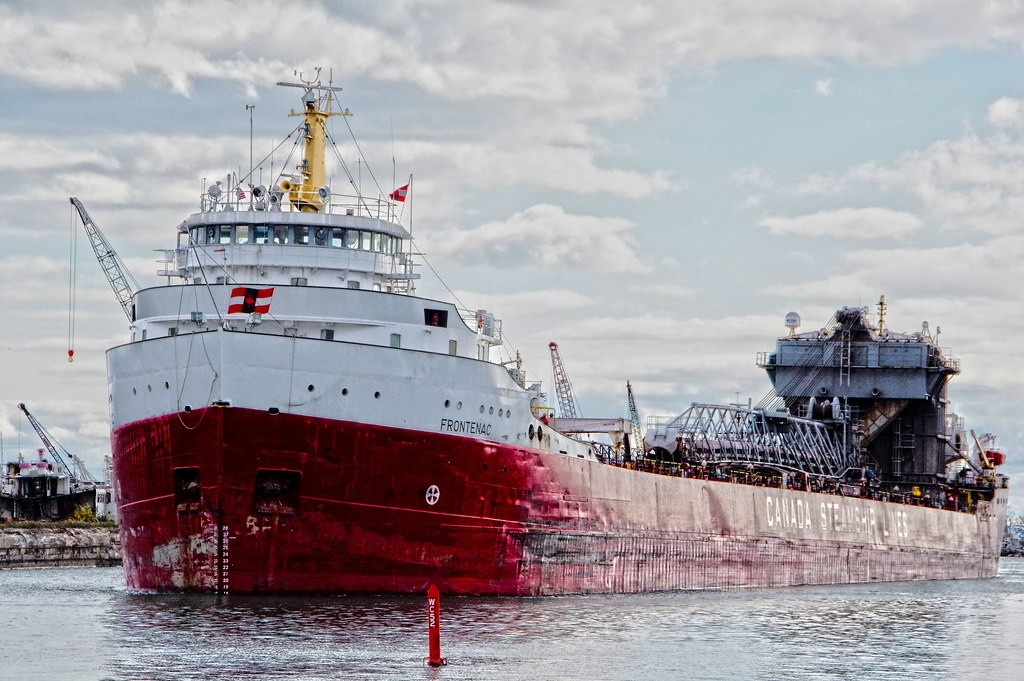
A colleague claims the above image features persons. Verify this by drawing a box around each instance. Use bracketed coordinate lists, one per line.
[670, 458, 980, 515]
[539, 412, 548, 426]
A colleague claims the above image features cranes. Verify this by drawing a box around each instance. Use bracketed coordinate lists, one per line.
[626, 380, 644, 457]
[549, 341, 590, 442]
[18, 403, 99, 490]
[68, 196, 142, 363]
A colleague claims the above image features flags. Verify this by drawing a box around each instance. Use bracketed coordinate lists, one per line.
[389, 184, 409, 203]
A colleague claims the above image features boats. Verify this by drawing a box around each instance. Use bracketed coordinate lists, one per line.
[0, 432, 96, 523]
[104, 66, 1010, 597]
